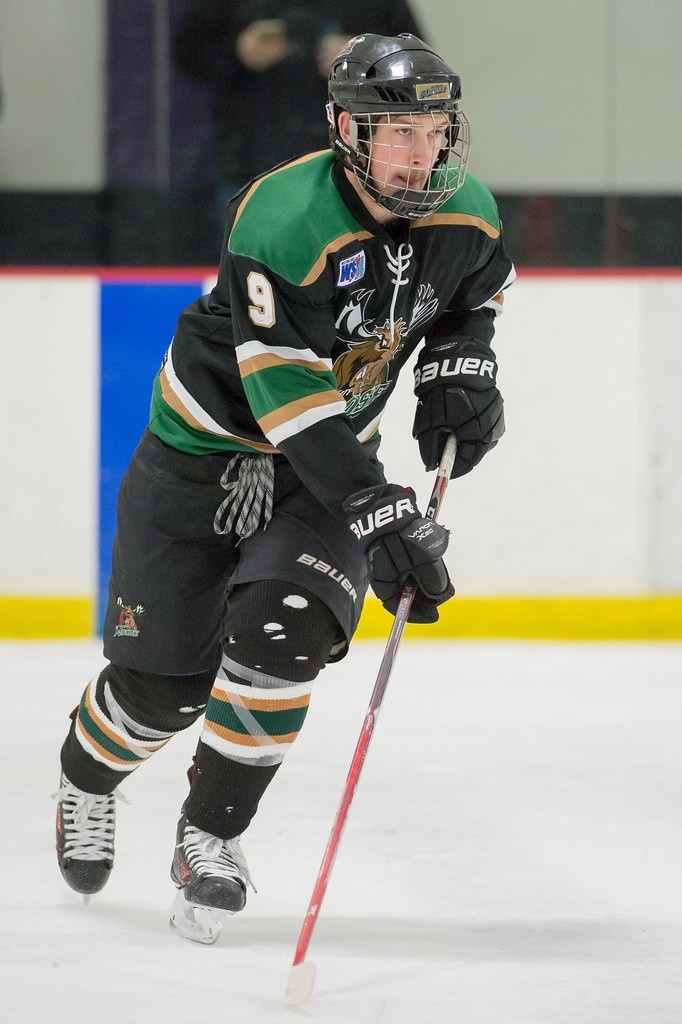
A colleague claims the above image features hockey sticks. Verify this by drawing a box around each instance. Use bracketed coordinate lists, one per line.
[288, 433, 460, 1009]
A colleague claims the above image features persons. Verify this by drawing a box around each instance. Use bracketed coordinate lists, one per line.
[53, 32, 519, 945]
[168, 0, 424, 267]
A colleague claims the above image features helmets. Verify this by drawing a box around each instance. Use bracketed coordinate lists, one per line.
[326, 31, 473, 221]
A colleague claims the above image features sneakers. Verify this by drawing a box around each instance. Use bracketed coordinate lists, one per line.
[50, 752, 131, 906]
[168, 810, 259, 948]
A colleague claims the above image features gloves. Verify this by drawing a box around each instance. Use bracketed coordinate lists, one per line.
[341, 484, 456, 625]
[411, 339, 506, 480]
